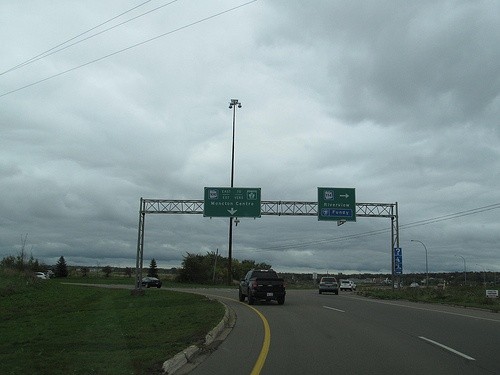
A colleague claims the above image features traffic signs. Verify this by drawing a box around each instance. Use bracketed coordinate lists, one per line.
[317, 186, 357, 222]
[203, 186, 261, 218]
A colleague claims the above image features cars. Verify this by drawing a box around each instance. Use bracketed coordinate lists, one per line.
[340, 279, 357, 292]
[136, 276, 162, 288]
[34, 270, 55, 280]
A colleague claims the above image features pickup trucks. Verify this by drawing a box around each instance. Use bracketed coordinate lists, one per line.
[238, 267, 286, 305]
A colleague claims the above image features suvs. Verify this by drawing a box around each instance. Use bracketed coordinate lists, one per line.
[318, 276, 341, 295]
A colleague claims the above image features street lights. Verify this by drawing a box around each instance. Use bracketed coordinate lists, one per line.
[410, 239, 429, 288]
[476, 264, 486, 284]
[227, 98, 242, 287]
[455, 254, 467, 286]
